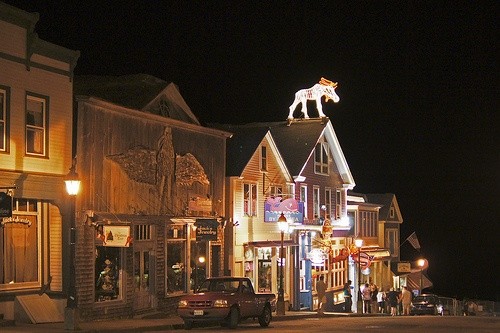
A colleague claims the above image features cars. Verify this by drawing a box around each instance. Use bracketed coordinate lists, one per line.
[409, 293, 464, 316]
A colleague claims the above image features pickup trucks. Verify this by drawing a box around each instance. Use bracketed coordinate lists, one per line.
[177, 276, 277, 328]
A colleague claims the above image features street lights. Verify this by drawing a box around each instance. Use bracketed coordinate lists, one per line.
[63, 159, 82, 331]
[276, 211, 289, 316]
[417, 259, 426, 294]
[354, 236, 364, 314]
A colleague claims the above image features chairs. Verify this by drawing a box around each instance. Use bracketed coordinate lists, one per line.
[218, 284, 226, 291]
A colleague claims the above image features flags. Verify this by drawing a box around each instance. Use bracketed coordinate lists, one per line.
[407, 233, 421, 249]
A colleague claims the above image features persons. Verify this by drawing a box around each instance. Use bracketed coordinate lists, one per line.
[361, 283, 412, 316]
[316, 276, 327, 317]
[344, 280, 353, 313]
